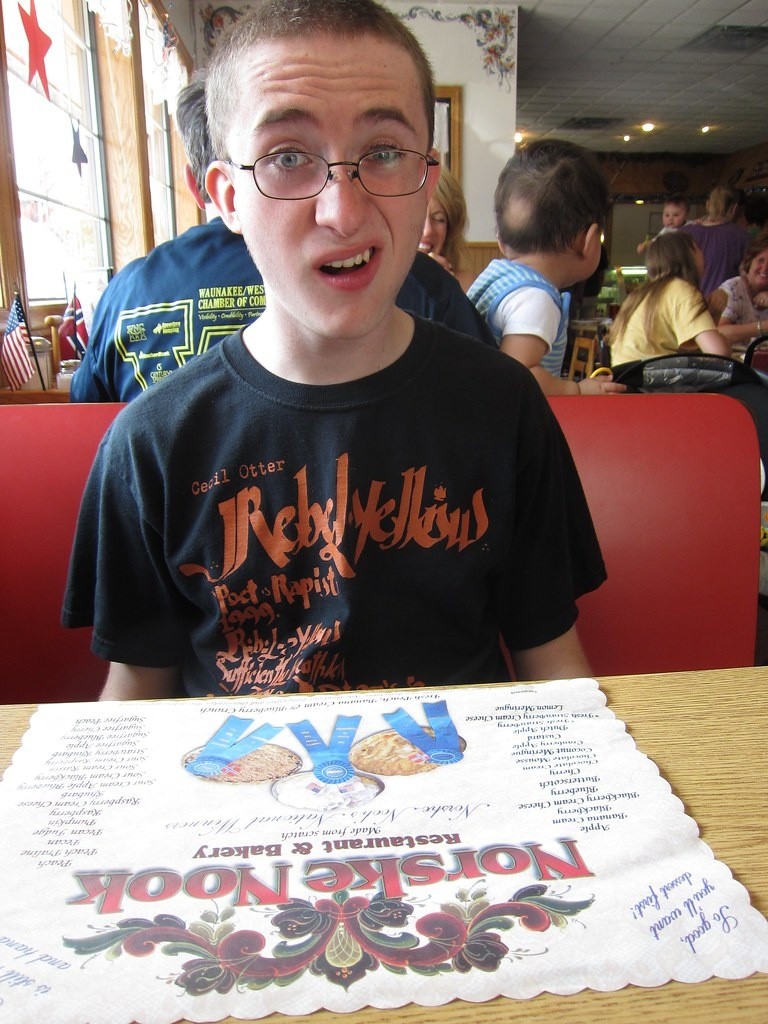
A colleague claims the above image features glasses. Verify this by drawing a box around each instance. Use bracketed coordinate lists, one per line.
[226, 148, 440, 201]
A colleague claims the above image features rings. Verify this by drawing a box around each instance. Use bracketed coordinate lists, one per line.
[760, 299, 764, 303]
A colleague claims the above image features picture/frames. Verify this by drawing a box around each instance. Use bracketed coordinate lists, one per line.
[432, 84, 463, 184]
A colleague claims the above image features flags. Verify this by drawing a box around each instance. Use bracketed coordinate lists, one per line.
[0, 295, 36, 391]
[58, 295, 89, 359]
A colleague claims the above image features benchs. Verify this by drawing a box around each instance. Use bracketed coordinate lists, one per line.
[0, 390, 760, 704]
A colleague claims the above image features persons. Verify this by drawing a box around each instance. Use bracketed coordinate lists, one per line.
[716, 234, 768, 347]
[608, 232, 730, 368]
[635, 184, 768, 300]
[416, 167, 466, 275]
[468, 137, 626, 395]
[71, 77, 497, 403]
[65, 0, 607, 703]
[559, 244, 607, 375]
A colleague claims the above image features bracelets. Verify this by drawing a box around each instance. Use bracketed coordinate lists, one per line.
[757, 321, 762, 337]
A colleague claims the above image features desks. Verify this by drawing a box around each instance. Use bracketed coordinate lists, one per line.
[0, 666, 768, 1023]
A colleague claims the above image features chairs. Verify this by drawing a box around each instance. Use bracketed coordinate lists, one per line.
[743, 333, 768, 372]
[568, 338, 601, 382]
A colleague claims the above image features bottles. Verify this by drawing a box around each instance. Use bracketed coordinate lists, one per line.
[22, 336, 81, 391]
